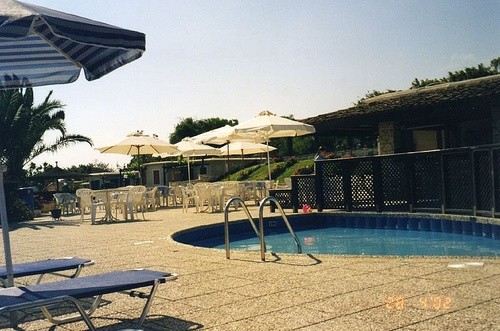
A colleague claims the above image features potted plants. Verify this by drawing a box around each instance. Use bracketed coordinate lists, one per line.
[49, 204, 61, 218]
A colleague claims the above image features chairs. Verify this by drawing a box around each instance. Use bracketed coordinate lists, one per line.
[117, 185, 146, 225]
[54, 192, 80, 216]
[147, 182, 182, 211]
[0, 255, 96, 288]
[242, 178, 279, 206]
[0, 267, 179, 331]
[77, 188, 95, 221]
[285, 178, 291, 188]
[184, 180, 247, 214]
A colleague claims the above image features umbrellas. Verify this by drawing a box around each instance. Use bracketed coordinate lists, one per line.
[29, 161, 93, 192]
[218, 115, 316, 189]
[152, 137, 224, 184]
[219, 141, 277, 175]
[94, 131, 179, 186]
[189, 124, 234, 182]
[0, 1, 145, 329]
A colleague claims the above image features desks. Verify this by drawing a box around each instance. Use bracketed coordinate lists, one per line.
[89, 188, 128, 223]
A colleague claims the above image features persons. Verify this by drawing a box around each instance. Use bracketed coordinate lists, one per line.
[313, 146, 328, 174]
